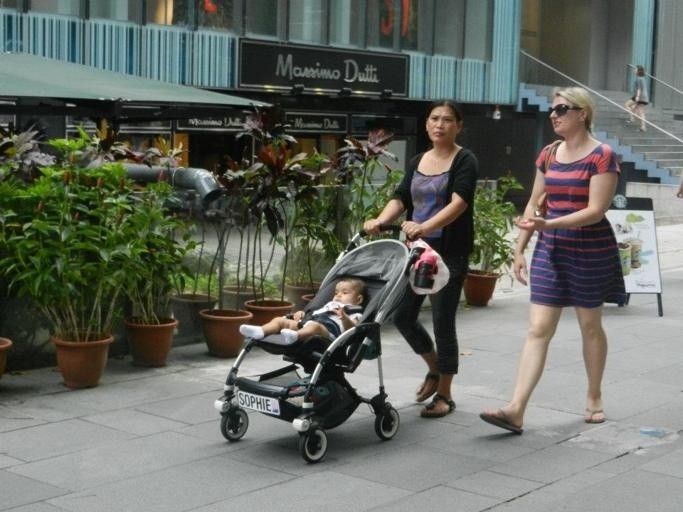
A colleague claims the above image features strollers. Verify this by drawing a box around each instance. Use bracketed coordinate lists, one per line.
[215, 222, 420, 468]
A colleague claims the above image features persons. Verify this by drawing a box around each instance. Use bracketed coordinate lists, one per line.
[239, 276, 369, 355]
[624, 64, 649, 132]
[363, 100, 478, 417]
[479, 87, 627, 435]
[677, 172, 683, 199]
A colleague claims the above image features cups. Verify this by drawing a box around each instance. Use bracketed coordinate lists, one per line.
[616, 242, 632, 276]
[624, 238, 643, 269]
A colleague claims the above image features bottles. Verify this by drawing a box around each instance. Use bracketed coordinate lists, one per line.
[413, 246, 439, 289]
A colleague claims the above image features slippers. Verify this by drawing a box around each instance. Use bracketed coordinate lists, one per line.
[421, 395, 456, 418]
[416, 372, 439, 402]
[585, 408, 605, 424]
[480, 407, 523, 434]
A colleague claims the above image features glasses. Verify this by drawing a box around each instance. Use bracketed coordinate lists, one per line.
[547, 103, 582, 116]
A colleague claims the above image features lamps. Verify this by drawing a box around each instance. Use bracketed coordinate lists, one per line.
[278, 84, 303, 102]
[371, 89, 393, 101]
[330, 88, 351, 102]
